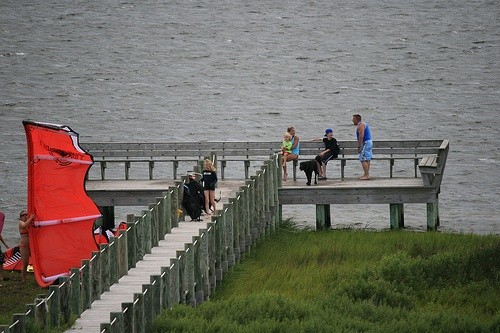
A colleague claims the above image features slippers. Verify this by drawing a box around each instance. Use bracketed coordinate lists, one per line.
[318, 175, 327, 179]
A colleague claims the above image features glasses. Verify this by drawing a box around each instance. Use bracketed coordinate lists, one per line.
[21, 214, 28, 216]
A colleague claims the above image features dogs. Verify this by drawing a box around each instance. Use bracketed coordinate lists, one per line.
[299, 155, 322, 186]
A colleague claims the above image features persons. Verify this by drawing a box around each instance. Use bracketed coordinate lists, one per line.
[281, 132, 292, 167]
[0, 235, 10, 287]
[313, 129, 340, 180]
[19, 211, 35, 283]
[200, 158, 218, 215]
[352, 115, 373, 179]
[282, 125, 299, 180]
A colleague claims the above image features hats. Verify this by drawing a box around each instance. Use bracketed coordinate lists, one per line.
[325, 129, 332, 134]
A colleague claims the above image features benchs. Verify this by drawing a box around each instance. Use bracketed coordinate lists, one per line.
[80, 139, 449, 187]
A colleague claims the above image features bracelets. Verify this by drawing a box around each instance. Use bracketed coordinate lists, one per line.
[358, 146, 361, 148]
